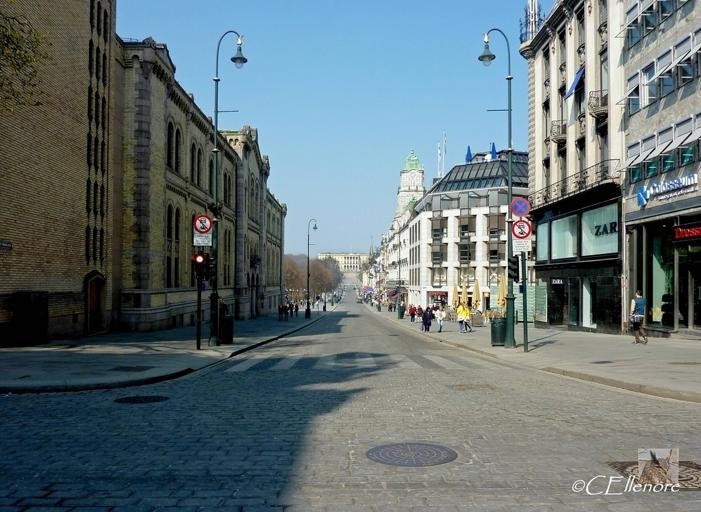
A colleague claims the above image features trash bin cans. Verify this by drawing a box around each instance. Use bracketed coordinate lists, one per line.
[398, 306, 405, 319]
[371, 303, 373, 306]
[211, 315, 234, 344]
[489, 317, 507, 347]
[378, 307, 381, 311]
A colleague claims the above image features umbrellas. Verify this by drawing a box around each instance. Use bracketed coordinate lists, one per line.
[471, 279, 480, 310]
[452, 285, 459, 307]
[461, 281, 469, 304]
[497, 273, 506, 308]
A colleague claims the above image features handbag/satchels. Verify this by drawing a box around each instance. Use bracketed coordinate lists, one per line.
[631, 315, 639, 323]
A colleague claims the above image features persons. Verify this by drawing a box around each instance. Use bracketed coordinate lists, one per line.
[388, 300, 396, 312]
[400, 300, 482, 333]
[629, 290, 648, 344]
[278, 303, 298, 321]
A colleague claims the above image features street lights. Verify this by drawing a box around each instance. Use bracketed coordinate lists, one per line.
[208, 29, 249, 338]
[305, 217, 318, 319]
[478, 28, 517, 350]
[389, 219, 401, 311]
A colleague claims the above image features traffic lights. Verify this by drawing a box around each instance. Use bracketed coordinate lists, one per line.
[193, 249, 205, 282]
